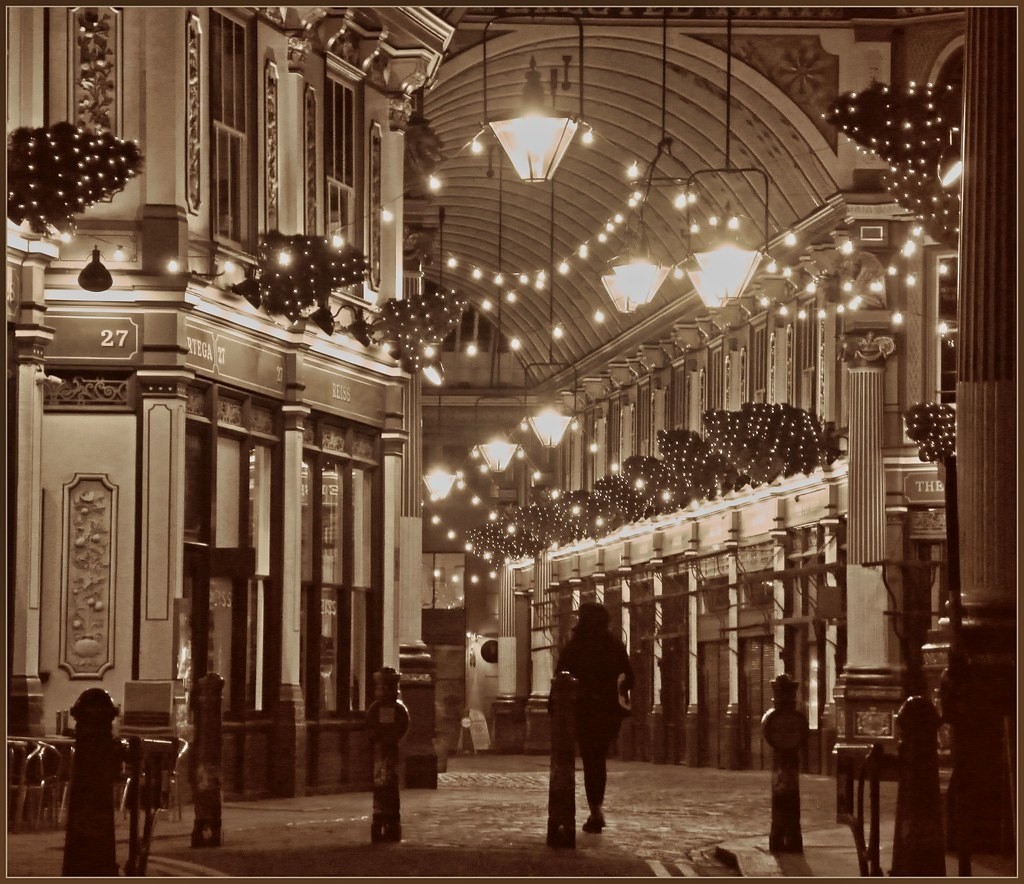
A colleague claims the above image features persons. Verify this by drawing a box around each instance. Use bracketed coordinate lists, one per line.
[547, 602, 635, 833]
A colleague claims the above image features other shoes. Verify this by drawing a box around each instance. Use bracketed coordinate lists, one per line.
[583, 815, 606, 834]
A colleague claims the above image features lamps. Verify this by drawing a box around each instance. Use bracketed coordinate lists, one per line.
[308, 297, 371, 348]
[422, 206, 457, 502]
[936, 124, 963, 189]
[524, 66, 578, 448]
[233, 264, 261, 310]
[482, 8, 584, 185]
[473, 144, 523, 474]
[77, 244, 113, 293]
[600, 6, 772, 314]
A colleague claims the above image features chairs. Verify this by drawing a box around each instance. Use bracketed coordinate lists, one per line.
[6, 734, 188, 835]
